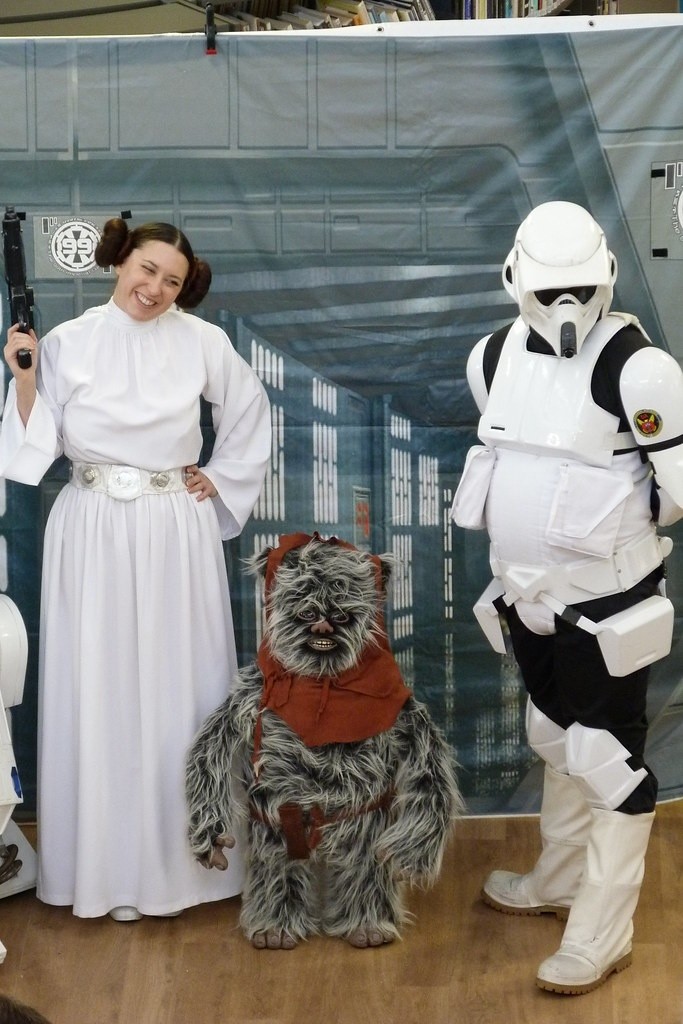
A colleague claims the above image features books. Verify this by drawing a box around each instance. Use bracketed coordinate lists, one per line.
[214, 0, 620, 31]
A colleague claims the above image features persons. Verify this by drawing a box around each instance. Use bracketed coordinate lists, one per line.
[450, 200, 683, 994]
[0, 218, 271, 921]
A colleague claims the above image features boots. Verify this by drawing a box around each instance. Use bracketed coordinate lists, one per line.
[536, 807, 656, 994]
[481, 762, 590, 921]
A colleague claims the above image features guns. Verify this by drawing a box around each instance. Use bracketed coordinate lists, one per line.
[1, 204, 37, 370]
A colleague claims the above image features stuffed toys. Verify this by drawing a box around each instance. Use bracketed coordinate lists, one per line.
[185, 530, 466, 950]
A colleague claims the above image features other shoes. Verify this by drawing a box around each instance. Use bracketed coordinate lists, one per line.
[109, 906, 143, 921]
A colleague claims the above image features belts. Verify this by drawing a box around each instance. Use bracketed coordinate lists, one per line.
[69, 461, 194, 502]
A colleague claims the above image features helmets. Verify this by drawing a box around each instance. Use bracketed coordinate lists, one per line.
[502, 201, 618, 357]
